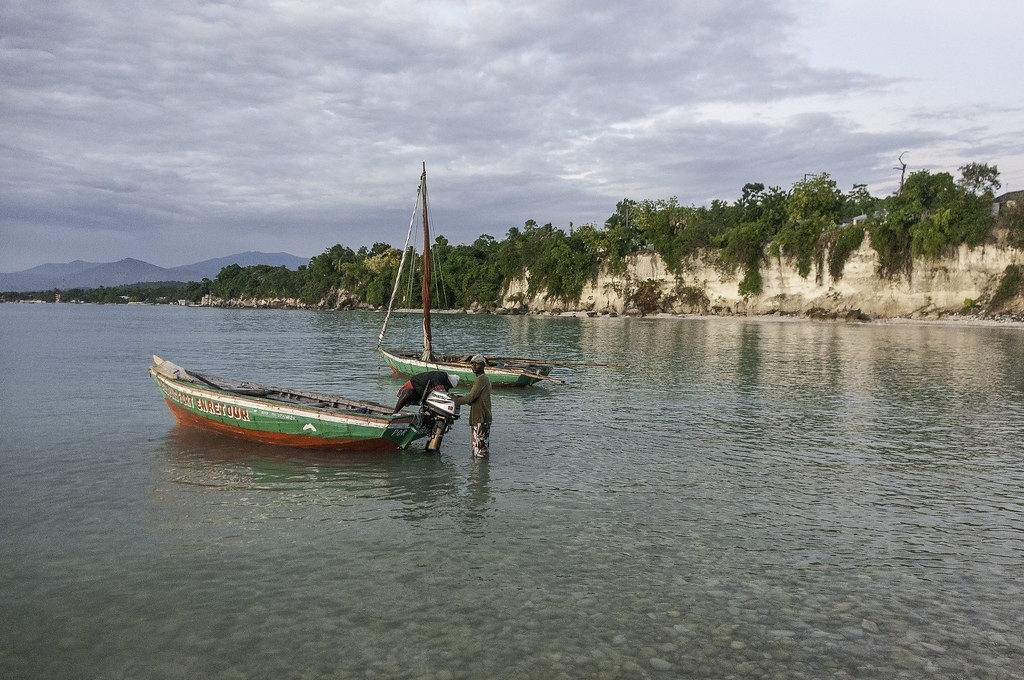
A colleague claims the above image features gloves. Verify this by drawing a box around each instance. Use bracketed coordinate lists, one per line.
[448, 393, 456, 400]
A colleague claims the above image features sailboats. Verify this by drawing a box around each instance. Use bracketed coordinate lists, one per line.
[378, 162, 608, 391]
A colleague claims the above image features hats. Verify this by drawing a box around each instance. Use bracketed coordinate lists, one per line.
[448, 374, 460, 389]
[470, 354, 486, 366]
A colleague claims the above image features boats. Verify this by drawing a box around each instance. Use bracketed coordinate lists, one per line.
[148, 354, 461, 458]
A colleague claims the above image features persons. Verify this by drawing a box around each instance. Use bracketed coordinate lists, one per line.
[448, 354, 492, 460]
[392, 371, 460, 414]
